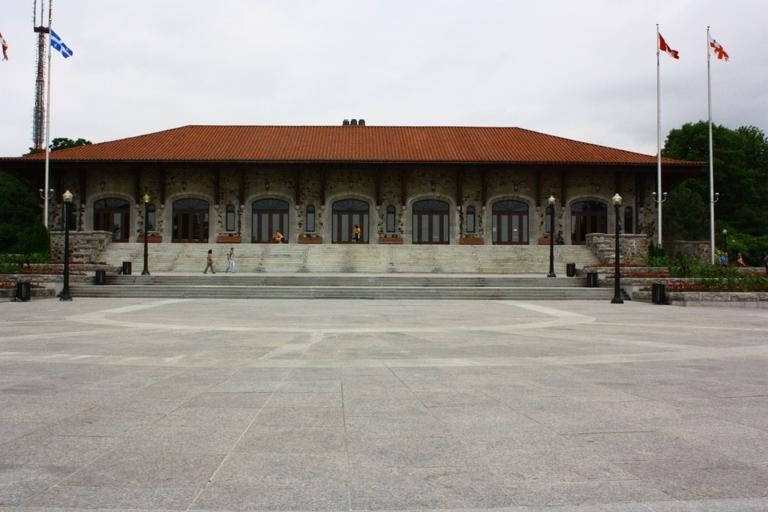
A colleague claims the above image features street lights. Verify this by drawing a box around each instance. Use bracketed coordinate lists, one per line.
[611, 193, 624, 304]
[142, 193, 151, 275]
[548, 195, 557, 277]
[59, 189, 74, 301]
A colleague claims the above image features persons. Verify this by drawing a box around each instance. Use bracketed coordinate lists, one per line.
[226, 248, 235, 272]
[274, 231, 285, 243]
[353, 225, 360, 242]
[720, 251, 728, 267]
[737, 252, 745, 266]
[203, 249, 216, 274]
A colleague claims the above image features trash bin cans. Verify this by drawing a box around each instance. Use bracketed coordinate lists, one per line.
[587, 273, 597, 287]
[123, 262, 131, 274]
[96, 270, 105, 284]
[17, 282, 30, 300]
[567, 264, 575, 276]
[652, 284, 665, 303]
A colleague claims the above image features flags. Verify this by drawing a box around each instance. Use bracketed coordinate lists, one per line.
[658, 32, 680, 60]
[710, 39, 729, 60]
[51, 30, 73, 58]
[0, 32, 9, 61]
